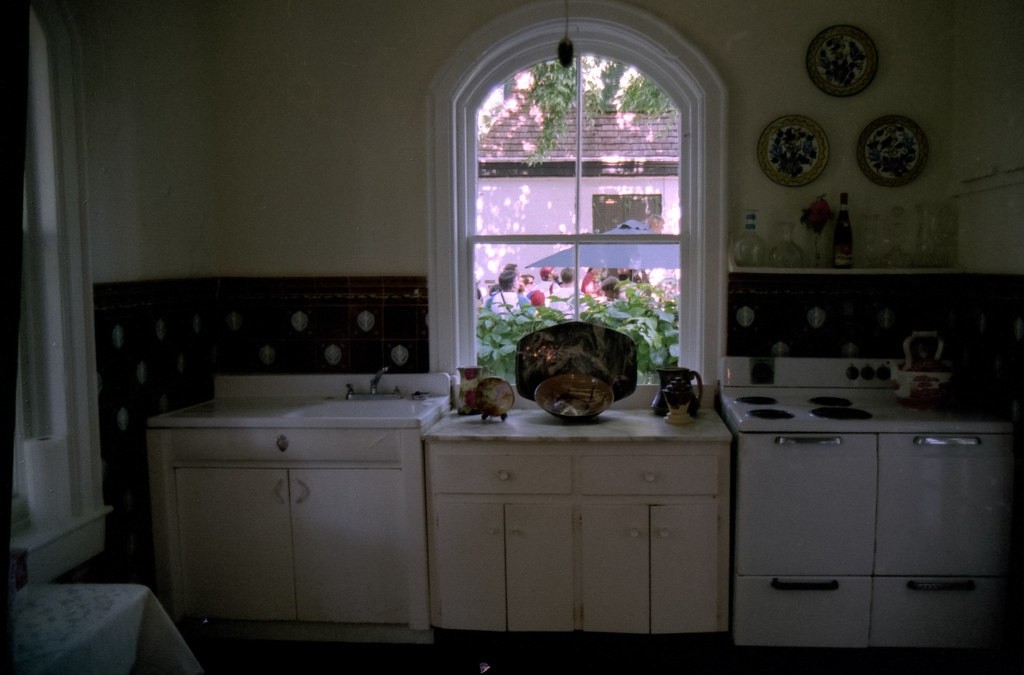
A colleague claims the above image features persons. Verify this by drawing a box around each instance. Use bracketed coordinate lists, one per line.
[475, 264, 670, 323]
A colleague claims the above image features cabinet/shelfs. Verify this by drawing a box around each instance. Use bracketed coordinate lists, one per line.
[425, 441, 729, 633]
[169, 465, 411, 623]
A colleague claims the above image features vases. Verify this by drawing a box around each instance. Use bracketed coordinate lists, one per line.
[792, 216, 836, 267]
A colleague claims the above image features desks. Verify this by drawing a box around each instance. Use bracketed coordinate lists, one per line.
[12, 506, 113, 585]
[9, 583, 150, 675]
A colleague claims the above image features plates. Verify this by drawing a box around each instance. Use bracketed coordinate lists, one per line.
[805, 23, 876, 96]
[758, 115, 827, 187]
[856, 114, 927, 186]
[515, 322, 638, 403]
[476, 377, 513, 416]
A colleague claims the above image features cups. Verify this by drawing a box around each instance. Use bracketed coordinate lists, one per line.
[857, 205, 953, 268]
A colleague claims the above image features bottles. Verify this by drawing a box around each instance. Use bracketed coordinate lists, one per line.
[732, 208, 766, 266]
[831, 192, 853, 269]
[769, 222, 805, 267]
[661, 377, 694, 424]
[454, 365, 482, 415]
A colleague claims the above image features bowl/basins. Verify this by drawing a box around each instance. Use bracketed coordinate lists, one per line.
[534, 372, 614, 422]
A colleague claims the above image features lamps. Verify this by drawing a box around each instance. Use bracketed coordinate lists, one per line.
[557, 0, 575, 67]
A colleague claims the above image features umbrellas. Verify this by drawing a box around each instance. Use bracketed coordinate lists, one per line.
[525, 219, 680, 270]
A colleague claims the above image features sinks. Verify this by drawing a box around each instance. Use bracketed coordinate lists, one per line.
[283, 401, 429, 419]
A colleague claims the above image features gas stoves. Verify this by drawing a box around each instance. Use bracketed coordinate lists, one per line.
[719, 356, 1013, 436]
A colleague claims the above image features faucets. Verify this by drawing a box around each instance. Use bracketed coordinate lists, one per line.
[345, 368, 403, 400]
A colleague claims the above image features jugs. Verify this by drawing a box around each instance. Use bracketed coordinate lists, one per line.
[653, 367, 704, 415]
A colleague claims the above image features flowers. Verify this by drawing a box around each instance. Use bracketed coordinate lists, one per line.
[799, 192, 836, 267]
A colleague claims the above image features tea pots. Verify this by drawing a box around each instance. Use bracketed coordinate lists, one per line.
[883, 332, 965, 416]
